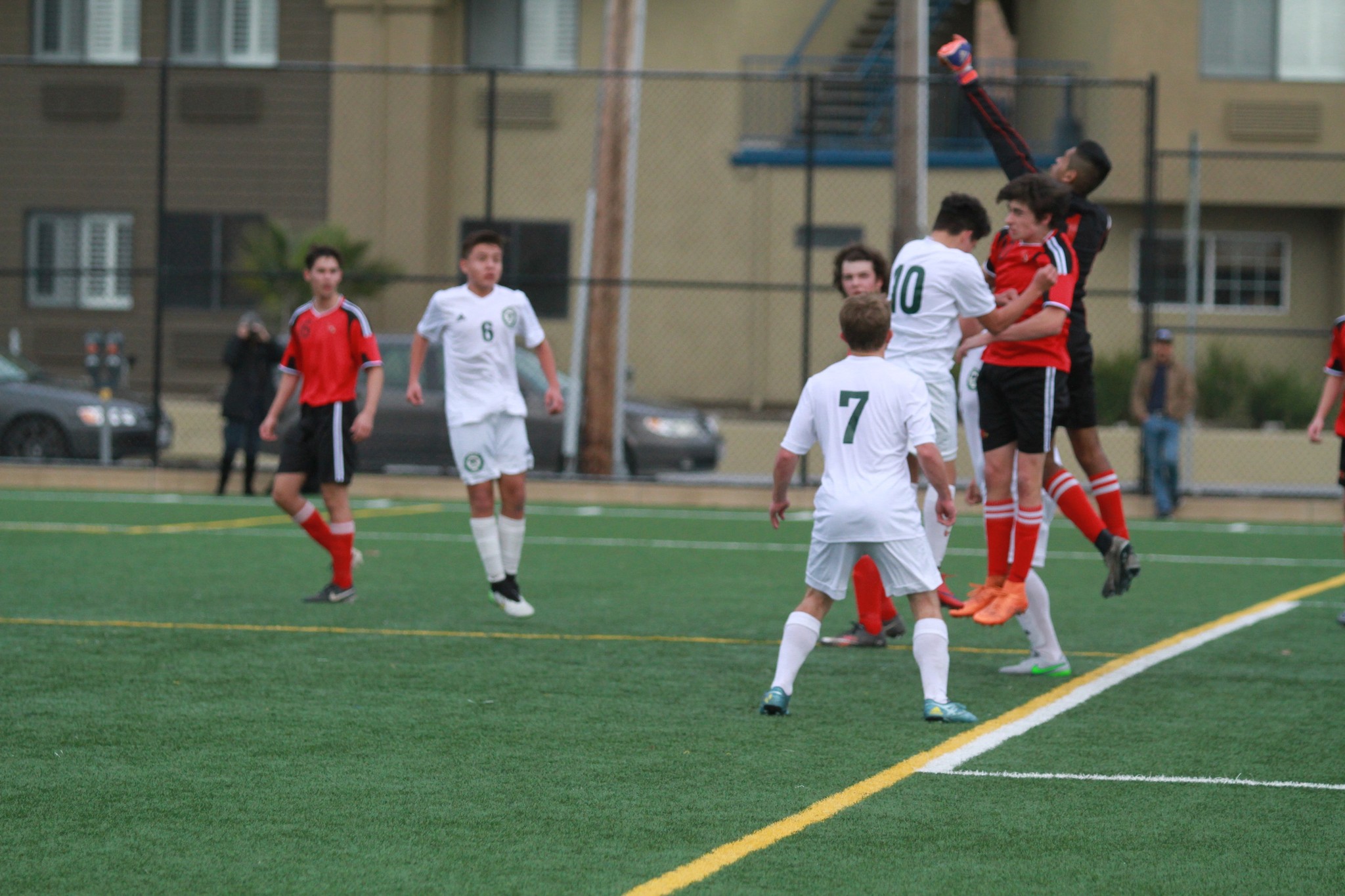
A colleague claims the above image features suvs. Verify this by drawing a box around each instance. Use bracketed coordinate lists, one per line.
[259, 331, 726, 481]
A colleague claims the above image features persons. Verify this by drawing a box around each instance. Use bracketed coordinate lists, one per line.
[817, 33, 1142, 679]
[257, 245, 385, 602]
[757, 292, 978, 722]
[1308, 315, 1345, 623]
[214, 312, 285, 496]
[1129, 328, 1194, 519]
[405, 230, 566, 618]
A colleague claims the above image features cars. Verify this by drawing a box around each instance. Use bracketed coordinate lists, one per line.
[0, 349, 178, 463]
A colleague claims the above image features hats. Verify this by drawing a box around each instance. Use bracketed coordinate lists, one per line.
[1154, 329, 1173, 343]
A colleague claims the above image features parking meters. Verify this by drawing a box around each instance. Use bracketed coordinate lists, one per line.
[84, 333, 131, 472]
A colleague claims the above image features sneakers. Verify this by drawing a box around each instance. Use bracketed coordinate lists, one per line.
[487, 589, 534, 619]
[306, 584, 353, 602]
[934, 578, 965, 611]
[924, 699, 979, 723]
[1104, 536, 1131, 592]
[973, 591, 1028, 627]
[999, 655, 1070, 677]
[759, 687, 793, 715]
[949, 583, 1002, 618]
[1102, 554, 1142, 598]
[820, 622, 884, 649]
[884, 615, 904, 636]
